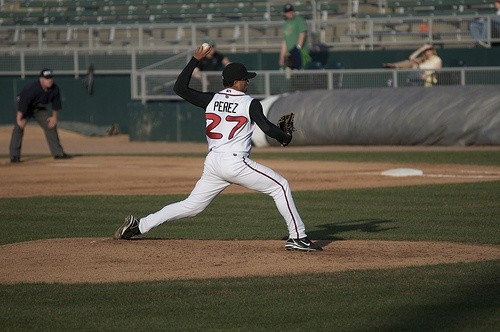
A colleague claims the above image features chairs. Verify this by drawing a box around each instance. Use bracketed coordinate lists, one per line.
[0, 0, 500, 49]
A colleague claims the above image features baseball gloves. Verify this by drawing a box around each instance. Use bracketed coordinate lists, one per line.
[276, 112, 296, 148]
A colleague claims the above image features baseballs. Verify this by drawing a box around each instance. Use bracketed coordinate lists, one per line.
[201, 42, 211, 52]
